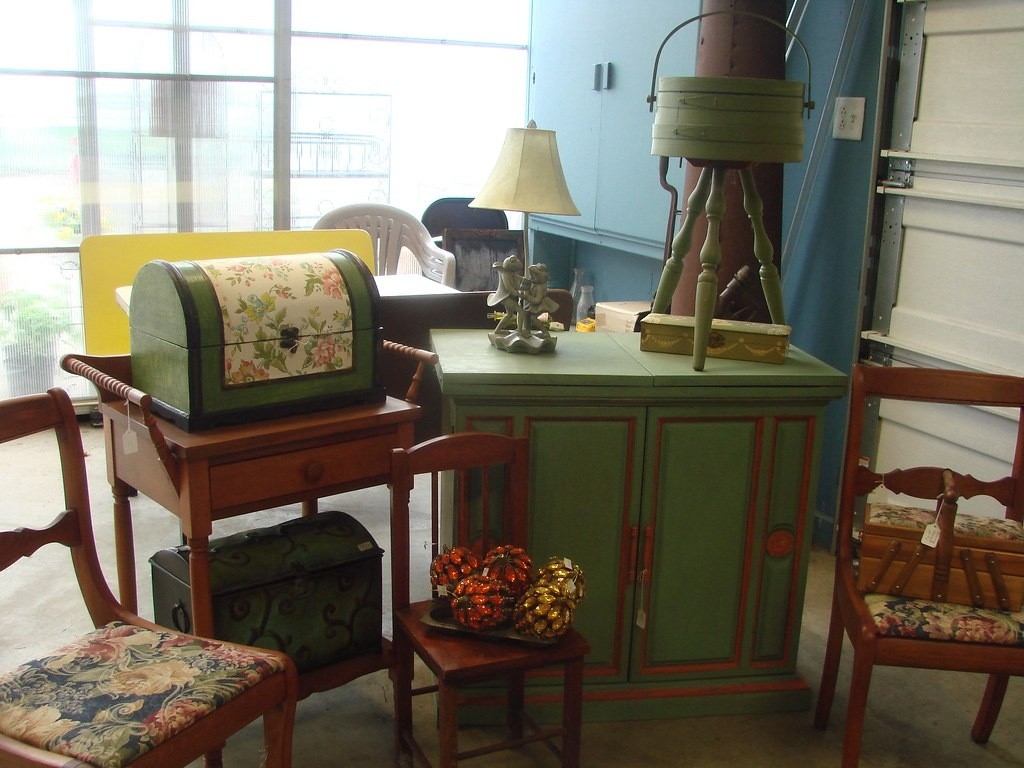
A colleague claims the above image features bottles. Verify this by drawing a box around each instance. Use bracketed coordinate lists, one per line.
[569, 268, 584, 325]
[576, 285, 595, 322]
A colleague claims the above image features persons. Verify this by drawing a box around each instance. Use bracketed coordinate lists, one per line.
[487, 254, 561, 338]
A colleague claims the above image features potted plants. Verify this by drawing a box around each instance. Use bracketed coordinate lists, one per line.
[0, 290, 82, 397]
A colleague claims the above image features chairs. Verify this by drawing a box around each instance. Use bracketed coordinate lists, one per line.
[0, 387, 298, 768]
[314, 204, 456, 289]
[815, 364, 1024, 768]
[392, 433, 592, 768]
[421, 198, 508, 237]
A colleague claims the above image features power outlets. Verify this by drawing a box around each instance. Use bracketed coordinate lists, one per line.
[832, 97, 866, 140]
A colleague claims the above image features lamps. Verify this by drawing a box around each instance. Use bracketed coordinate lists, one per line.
[468, 120, 582, 355]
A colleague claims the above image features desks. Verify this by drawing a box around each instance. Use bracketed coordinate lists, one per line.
[60, 339, 439, 768]
[115, 274, 574, 443]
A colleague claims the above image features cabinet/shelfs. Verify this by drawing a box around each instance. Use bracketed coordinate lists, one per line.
[526, 0, 892, 549]
[430, 329, 848, 730]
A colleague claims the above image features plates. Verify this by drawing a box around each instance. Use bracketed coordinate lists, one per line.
[420, 601, 560, 646]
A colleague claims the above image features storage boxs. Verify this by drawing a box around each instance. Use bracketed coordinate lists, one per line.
[640, 314, 792, 365]
[850, 469, 1023, 610]
[150, 510, 386, 670]
[595, 301, 652, 333]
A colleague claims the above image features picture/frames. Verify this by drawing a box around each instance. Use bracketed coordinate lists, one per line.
[443, 228, 524, 292]
